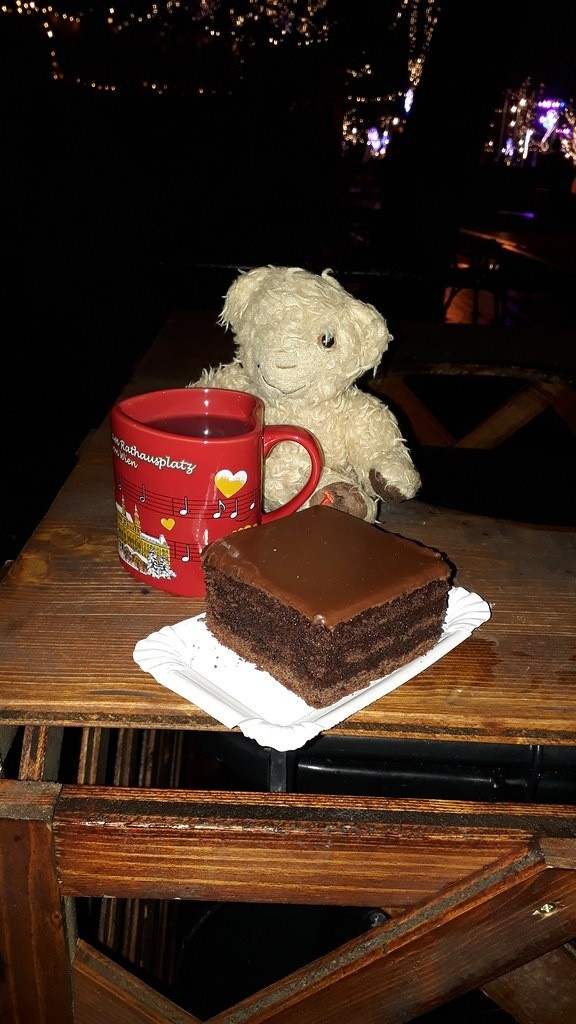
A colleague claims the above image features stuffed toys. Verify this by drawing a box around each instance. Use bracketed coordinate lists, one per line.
[183, 263, 420, 527]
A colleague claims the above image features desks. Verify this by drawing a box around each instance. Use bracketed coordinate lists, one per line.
[0, 379, 574, 1024]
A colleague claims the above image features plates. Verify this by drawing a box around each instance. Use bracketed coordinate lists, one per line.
[132, 585, 490, 753]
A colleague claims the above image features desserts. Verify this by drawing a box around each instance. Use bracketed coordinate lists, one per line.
[199, 505, 454, 709]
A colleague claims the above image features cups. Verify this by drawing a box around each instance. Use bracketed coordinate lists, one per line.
[109, 388, 324, 599]
